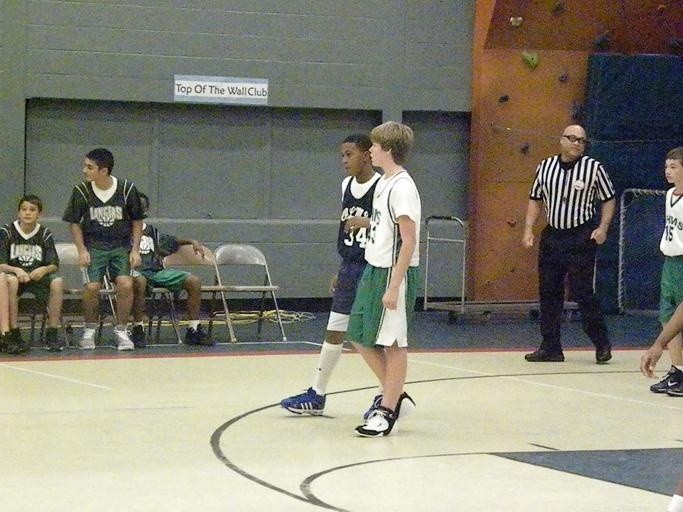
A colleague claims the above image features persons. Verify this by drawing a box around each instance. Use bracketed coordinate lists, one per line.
[0, 192, 63, 354]
[646, 146, 682, 397]
[277, 132, 406, 419]
[125, 187, 216, 349]
[341, 118, 423, 437]
[57, 146, 143, 352]
[638, 298, 682, 379]
[0, 228, 19, 357]
[517, 122, 614, 365]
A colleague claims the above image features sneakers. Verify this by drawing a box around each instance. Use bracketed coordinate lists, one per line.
[650, 364, 683, 396]
[0, 327, 29, 354]
[281, 387, 327, 416]
[525, 347, 565, 361]
[112, 324, 146, 350]
[184, 324, 216, 346]
[596, 342, 612, 363]
[78, 322, 98, 350]
[44, 328, 63, 352]
[354, 391, 417, 438]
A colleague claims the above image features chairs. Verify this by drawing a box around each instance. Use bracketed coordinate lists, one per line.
[99, 238, 182, 344]
[209, 243, 288, 341]
[11, 290, 47, 347]
[45, 242, 121, 345]
[162, 241, 236, 344]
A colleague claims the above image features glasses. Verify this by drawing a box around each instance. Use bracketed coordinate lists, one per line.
[563, 134, 586, 144]
